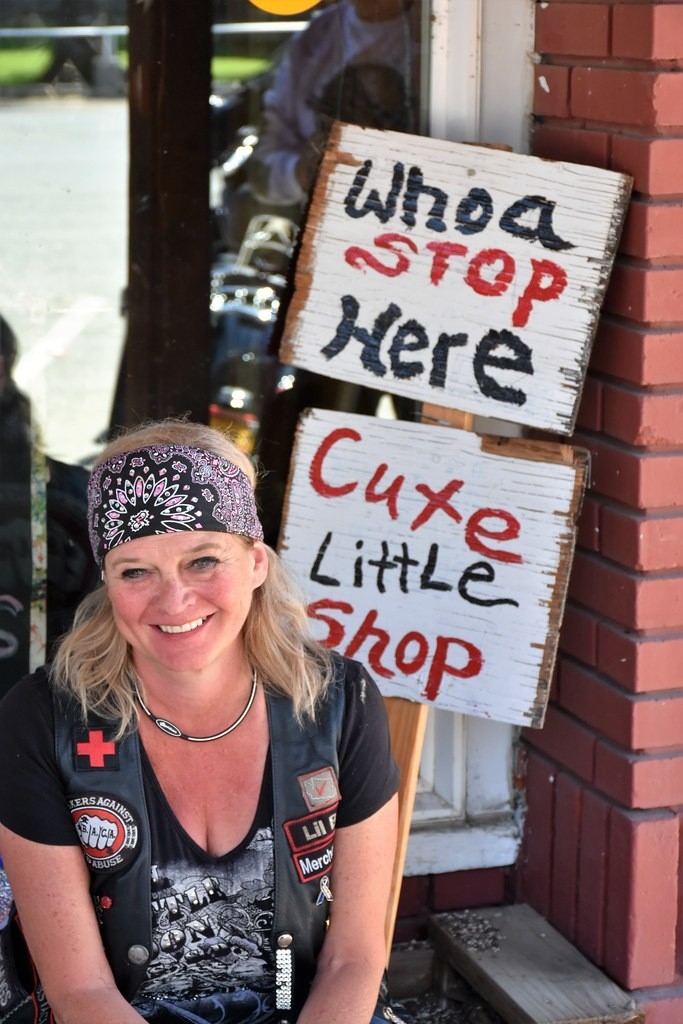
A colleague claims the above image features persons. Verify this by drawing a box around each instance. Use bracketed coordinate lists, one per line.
[0, 423, 399, 1024]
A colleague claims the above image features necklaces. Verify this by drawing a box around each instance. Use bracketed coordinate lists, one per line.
[135, 665, 257, 742]
